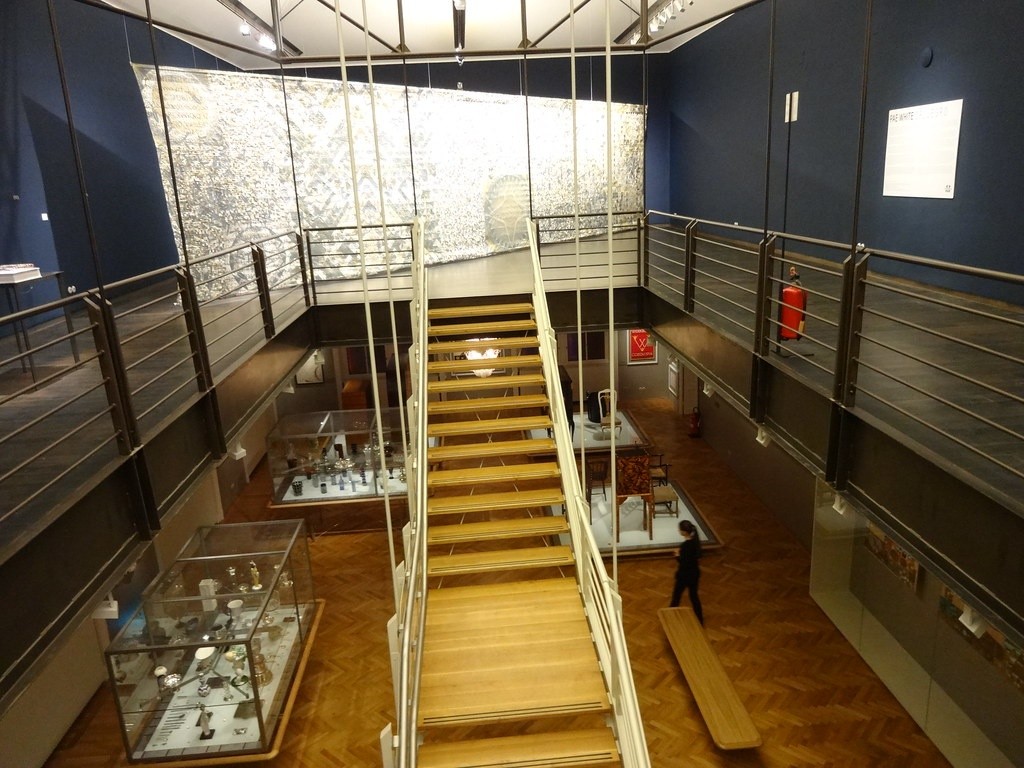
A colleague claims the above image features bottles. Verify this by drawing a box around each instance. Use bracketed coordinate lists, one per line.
[287, 443, 298, 469]
[339, 476, 344, 490]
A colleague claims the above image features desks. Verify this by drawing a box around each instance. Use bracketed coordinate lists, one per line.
[0, 270, 81, 392]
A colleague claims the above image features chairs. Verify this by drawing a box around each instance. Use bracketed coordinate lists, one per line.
[646, 452, 671, 488]
[611, 446, 655, 543]
[648, 463, 680, 519]
[585, 459, 609, 501]
[598, 389, 623, 440]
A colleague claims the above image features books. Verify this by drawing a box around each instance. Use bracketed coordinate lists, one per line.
[0, 267, 43, 284]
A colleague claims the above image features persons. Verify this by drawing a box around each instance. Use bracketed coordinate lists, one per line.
[669, 520, 705, 624]
[684, 407, 701, 438]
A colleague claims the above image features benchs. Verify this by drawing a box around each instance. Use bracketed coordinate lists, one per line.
[656, 607, 763, 752]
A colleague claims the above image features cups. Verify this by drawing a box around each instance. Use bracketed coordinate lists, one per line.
[292, 481, 302, 496]
[261, 590, 280, 612]
[331, 475, 336, 485]
[321, 483, 326, 493]
[227, 600, 243, 619]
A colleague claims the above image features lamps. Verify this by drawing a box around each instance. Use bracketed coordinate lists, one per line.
[703, 381, 715, 398]
[647, 335, 656, 346]
[958, 604, 987, 638]
[230, 440, 247, 460]
[462, 337, 501, 378]
[832, 494, 851, 517]
[314, 351, 325, 365]
[92, 591, 119, 619]
[755, 428, 772, 447]
[283, 379, 295, 394]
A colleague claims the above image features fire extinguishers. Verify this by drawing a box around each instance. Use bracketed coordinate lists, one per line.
[690, 406, 701, 434]
[779, 267, 806, 341]
